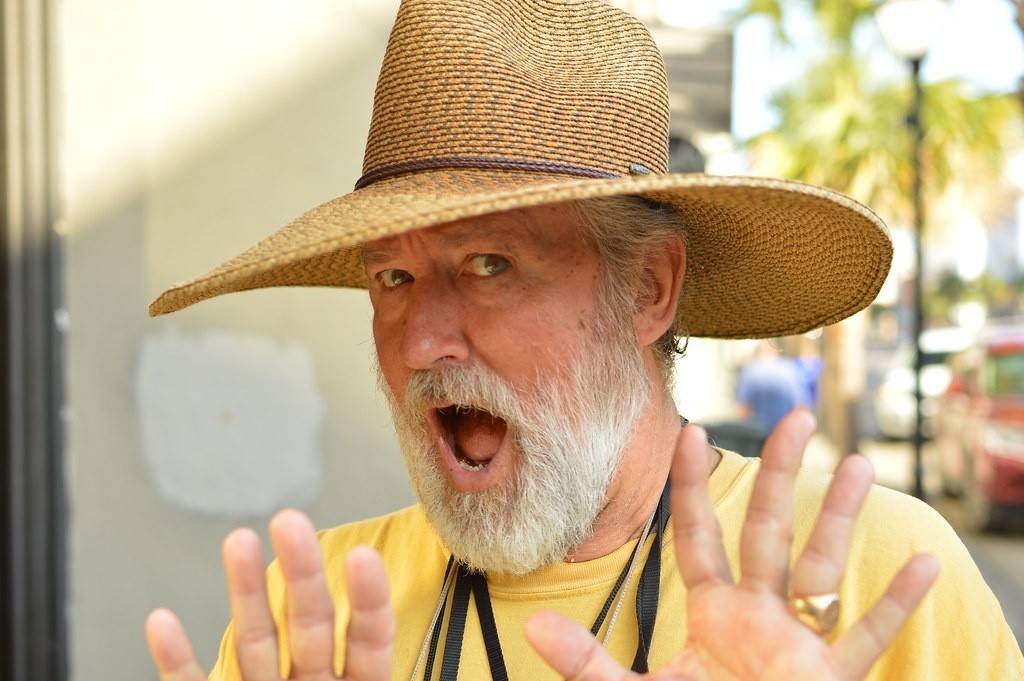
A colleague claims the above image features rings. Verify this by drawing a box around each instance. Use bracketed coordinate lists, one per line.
[787, 592, 840, 634]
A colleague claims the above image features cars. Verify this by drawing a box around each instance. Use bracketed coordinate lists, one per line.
[939, 327, 1024, 533]
[876, 331, 970, 442]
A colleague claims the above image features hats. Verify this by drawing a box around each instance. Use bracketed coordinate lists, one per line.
[149, 0, 892, 340]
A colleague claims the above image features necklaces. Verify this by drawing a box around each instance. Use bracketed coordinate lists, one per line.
[402, 470, 657, 681]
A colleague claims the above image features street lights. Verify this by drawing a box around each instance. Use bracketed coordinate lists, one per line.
[877, 0, 945, 498]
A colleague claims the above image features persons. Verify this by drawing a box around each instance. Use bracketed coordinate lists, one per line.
[734, 338, 809, 428]
[793, 338, 822, 398]
[145, 0, 1024, 681]
